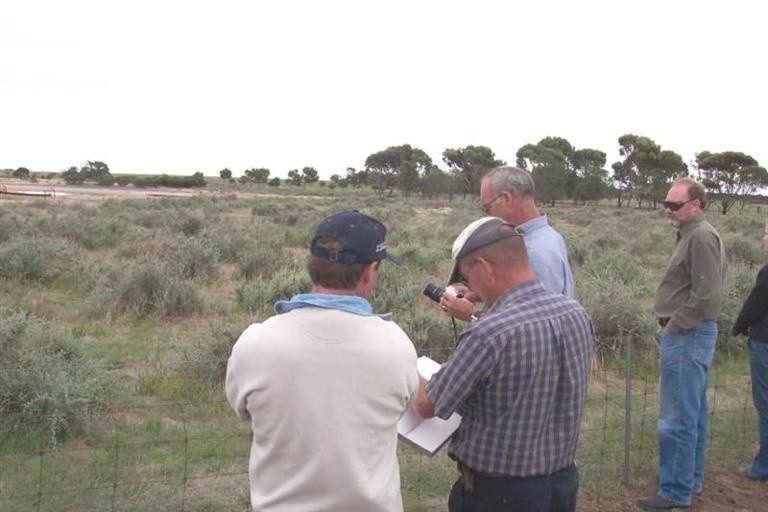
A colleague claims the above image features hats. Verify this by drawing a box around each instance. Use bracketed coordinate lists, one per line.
[311, 208, 403, 270]
[447, 217, 524, 287]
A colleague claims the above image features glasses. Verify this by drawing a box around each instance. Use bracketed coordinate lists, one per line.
[480, 192, 504, 214]
[660, 196, 697, 212]
[458, 259, 479, 291]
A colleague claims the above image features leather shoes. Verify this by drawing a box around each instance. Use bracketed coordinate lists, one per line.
[636, 492, 686, 512]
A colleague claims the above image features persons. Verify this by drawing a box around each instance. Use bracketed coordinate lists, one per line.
[414, 216, 596, 512]
[636, 179, 728, 512]
[440, 165, 574, 322]
[225, 210, 419, 512]
[730, 223, 768, 480]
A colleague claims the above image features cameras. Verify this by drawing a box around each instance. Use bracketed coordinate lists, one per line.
[424, 284, 464, 309]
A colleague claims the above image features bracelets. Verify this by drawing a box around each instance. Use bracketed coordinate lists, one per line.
[470, 311, 480, 324]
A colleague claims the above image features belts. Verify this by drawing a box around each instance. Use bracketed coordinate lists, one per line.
[658, 316, 675, 329]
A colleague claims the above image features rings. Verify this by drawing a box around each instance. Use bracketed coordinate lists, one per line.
[442, 306, 445, 309]
